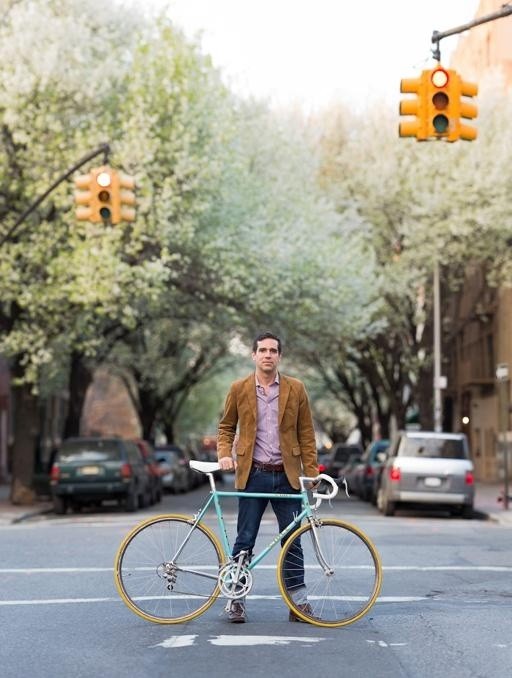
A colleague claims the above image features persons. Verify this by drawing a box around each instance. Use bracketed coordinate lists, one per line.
[217, 333, 323, 623]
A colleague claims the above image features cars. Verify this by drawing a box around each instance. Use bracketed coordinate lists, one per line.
[49, 437, 222, 515]
[316, 429, 475, 519]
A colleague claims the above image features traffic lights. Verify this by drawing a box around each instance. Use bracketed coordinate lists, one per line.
[399, 67, 479, 142]
[75, 166, 136, 225]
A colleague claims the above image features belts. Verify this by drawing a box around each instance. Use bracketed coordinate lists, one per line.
[252, 459, 284, 471]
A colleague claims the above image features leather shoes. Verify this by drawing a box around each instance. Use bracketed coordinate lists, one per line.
[227, 601, 246, 621]
[289, 603, 321, 622]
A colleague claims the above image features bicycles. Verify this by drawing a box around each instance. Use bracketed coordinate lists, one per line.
[113, 460, 383, 628]
